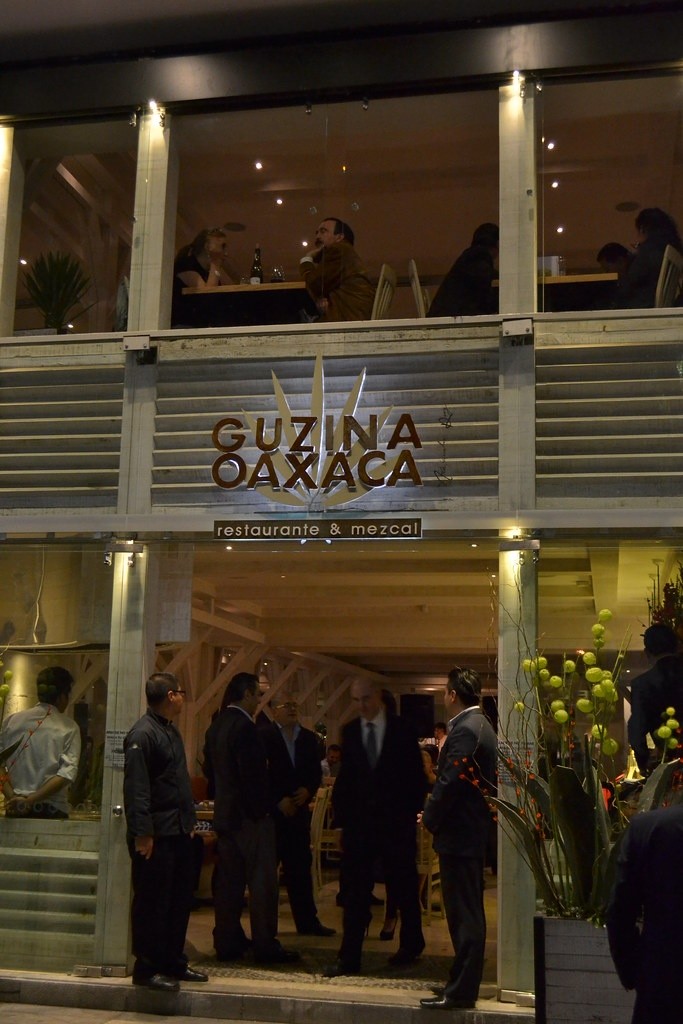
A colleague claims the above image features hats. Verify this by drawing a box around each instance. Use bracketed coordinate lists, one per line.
[421, 744, 440, 766]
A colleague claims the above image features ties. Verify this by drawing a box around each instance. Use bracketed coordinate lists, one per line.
[365, 722, 376, 762]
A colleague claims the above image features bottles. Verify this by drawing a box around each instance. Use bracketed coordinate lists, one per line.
[249, 243, 264, 284]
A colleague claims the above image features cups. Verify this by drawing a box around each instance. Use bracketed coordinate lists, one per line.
[270, 264, 286, 282]
[559, 255, 570, 276]
[239, 273, 250, 285]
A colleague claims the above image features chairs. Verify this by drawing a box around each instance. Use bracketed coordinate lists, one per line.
[401, 260, 432, 319]
[371, 264, 396, 319]
[304, 786, 446, 925]
[654, 245, 683, 309]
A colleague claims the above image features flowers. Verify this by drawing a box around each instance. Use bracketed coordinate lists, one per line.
[452, 598, 683, 919]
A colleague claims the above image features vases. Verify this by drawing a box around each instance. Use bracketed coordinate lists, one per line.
[543, 913, 636, 1024]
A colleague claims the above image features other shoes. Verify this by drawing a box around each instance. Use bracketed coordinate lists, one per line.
[369, 892, 385, 905]
[252, 938, 300, 964]
[387, 949, 417, 966]
[331, 958, 363, 977]
[216, 937, 261, 961]
[297, 917, 337, 936]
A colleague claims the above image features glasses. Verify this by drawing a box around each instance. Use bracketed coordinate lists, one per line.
[275, 703, 300, 709]
[173, 690, 186, 697]
[253, 691, 266, 697]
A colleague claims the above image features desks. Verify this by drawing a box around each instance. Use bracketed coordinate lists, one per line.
[490, 273, 619, 314]
[186, 281, 311, 326]
[194, 807, 213, 819]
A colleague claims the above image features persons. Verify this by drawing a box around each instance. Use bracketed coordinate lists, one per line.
[170, 229, 236, 330]
[596, 207, 680, 310]
[542, 702, 584, 785]
[299, 218, 377, 324]
[201, 672, 449, 978]
[424, 223, 499, 318]
[123, 672, 208, 991]
[418, 664, 498, 1010]
[605, 802, 683, 1024]
[0, 667, 81, 820]
[627, 624, 683, 771]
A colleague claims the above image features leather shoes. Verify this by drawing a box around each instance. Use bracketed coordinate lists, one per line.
[132, 973, 180, 992]
[161, 965, 209, 981]
[430, 986, 447, 995]
[419, 992, 476, 1009]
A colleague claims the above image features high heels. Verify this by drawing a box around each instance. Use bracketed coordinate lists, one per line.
[380, 913, 398, 941]
[364, 911, 374, 937]
